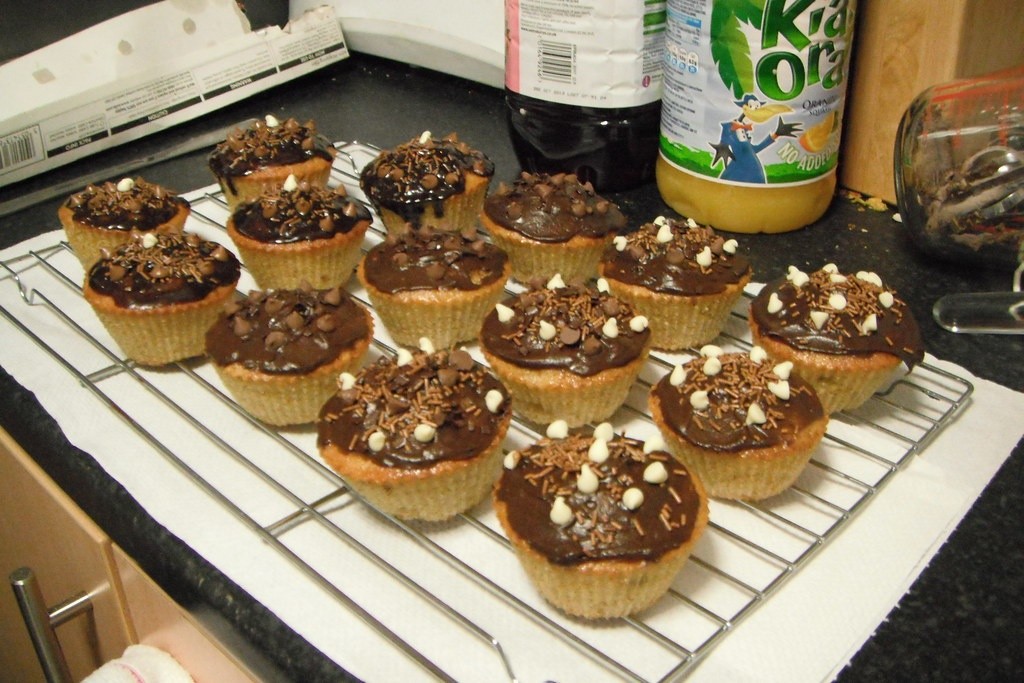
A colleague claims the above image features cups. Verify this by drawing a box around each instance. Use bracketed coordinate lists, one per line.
[896, 70, 1023, 270]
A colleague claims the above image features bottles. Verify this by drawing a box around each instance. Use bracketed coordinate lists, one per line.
[658, 0, 859, 236]
[503, 0, 670, 193]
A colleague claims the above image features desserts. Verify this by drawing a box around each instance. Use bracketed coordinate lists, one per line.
[55, 106, 924, 618]
